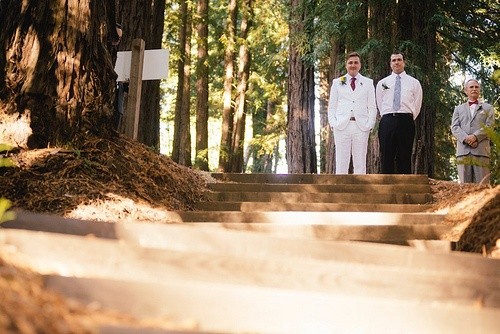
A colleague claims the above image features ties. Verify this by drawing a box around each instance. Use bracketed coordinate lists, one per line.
[351, 78, 356, 91]
[393, 75, 401, 111]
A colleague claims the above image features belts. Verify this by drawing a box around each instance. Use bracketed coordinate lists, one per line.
[350, 117, 356, 120]
[383, 113, 413, 118]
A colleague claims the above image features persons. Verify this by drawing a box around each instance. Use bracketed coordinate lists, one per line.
[328, 52, 377, 175]
[451, 79, 495, 184]
[376, 50, 423, 175]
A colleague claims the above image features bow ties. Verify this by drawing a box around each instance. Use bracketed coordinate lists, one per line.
[469, 100, 478, 106]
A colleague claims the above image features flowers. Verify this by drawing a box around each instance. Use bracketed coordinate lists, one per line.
[340, 76, 346, 85]
